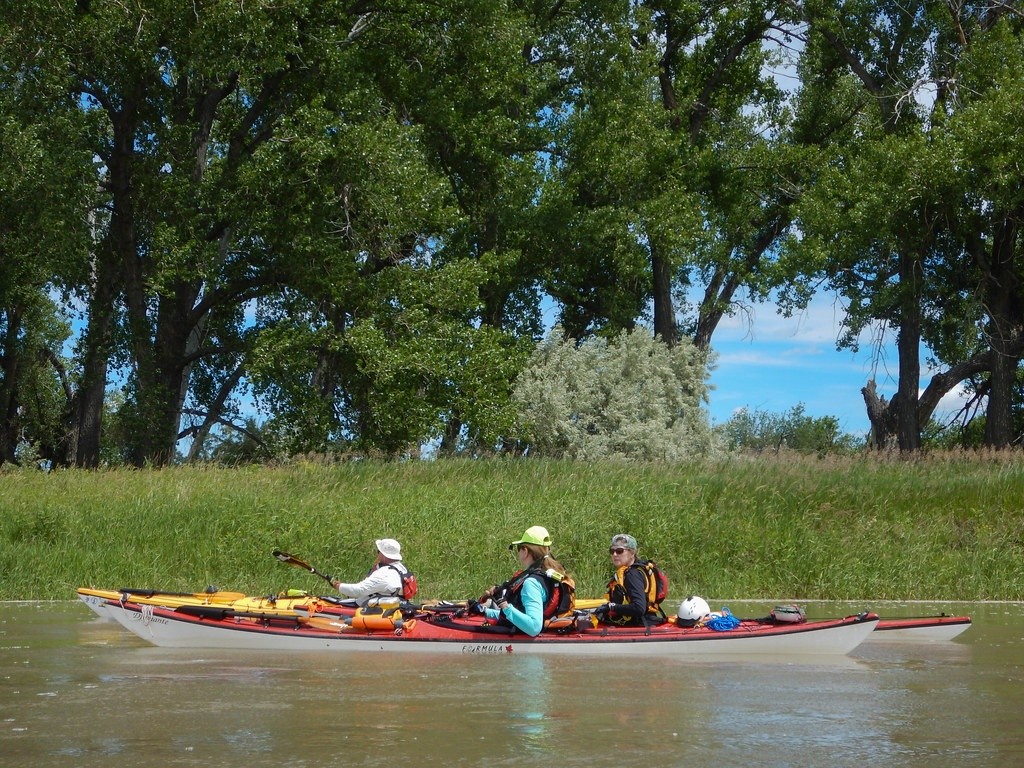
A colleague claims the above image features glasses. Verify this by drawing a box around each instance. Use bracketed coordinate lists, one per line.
[609, 548, 629, 555]
[378, 549, 381, 554]
[517, 545, 526, 552]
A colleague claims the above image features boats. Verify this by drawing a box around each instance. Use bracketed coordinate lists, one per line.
[77, 582, 624, 618]
[102, 598, 876, 653]
[866, 616, 972, 642]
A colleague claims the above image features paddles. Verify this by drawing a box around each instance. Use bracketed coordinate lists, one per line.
[477, 559, 542, 605]
[272, 551, 335, 583]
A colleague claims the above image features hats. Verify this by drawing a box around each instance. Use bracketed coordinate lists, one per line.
[611, 534, 637, 550]
[375, 538, 402, 560]
[511, 526, 552, 546]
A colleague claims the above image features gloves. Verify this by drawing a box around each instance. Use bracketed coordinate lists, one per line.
[594, 603, 610, 616]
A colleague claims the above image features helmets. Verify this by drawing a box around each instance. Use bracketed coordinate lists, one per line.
[677, 594, 710, 628]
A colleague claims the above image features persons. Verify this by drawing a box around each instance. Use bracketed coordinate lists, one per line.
[466, 524, 565, 637]
[592, 533, 656, 628]
[330, 538, 408, 598]
